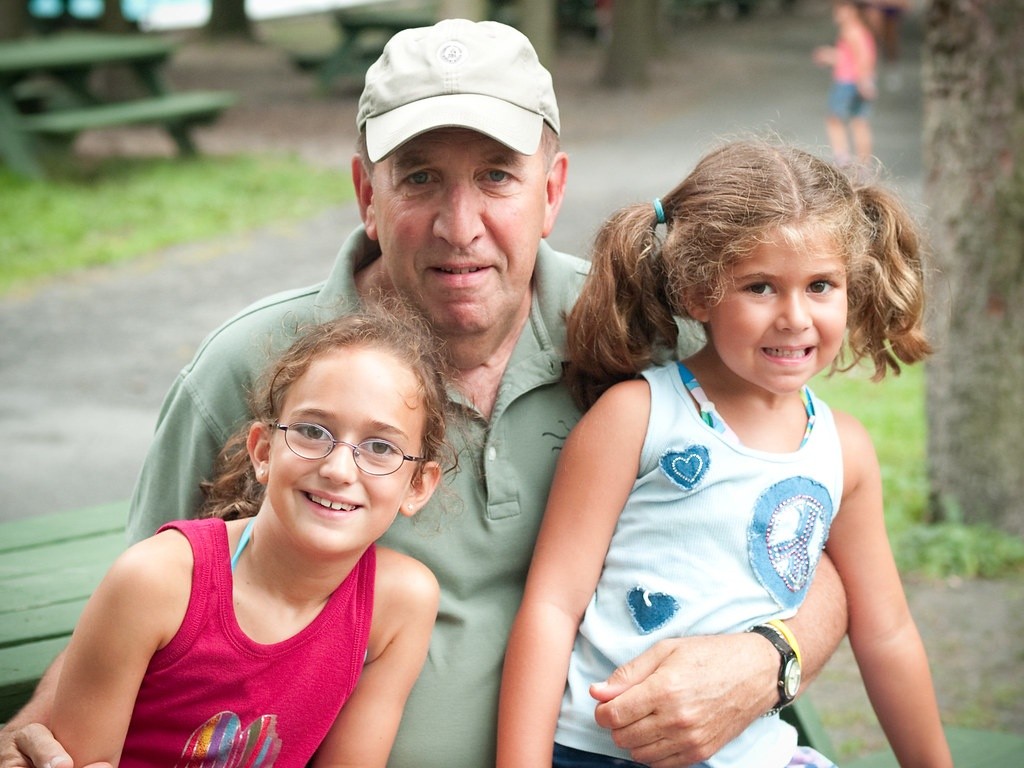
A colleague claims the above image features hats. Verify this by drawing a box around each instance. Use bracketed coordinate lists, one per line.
[355, 18, 560, 164]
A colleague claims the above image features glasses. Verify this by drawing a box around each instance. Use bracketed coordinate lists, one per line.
[269, 420, 430, 477]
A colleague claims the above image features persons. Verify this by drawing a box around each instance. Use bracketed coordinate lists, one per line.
[55, 282, 477, 768]
[810, 1, 909, 176]
[493, 133, 954, 768]
[0, 18, 849, 768]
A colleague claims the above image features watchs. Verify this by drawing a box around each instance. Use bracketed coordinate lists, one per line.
[747, 624, 801, 717]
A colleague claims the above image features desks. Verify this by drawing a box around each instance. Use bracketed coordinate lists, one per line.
[0, 39, 172, 125]
[332, 9, 440, 54]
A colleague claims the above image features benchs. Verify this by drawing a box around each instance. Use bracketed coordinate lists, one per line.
[0, 502, 194, 689]
[29, 92, 233, 163]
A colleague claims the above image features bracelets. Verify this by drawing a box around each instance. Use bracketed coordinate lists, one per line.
[764, 619, 802, 667]
